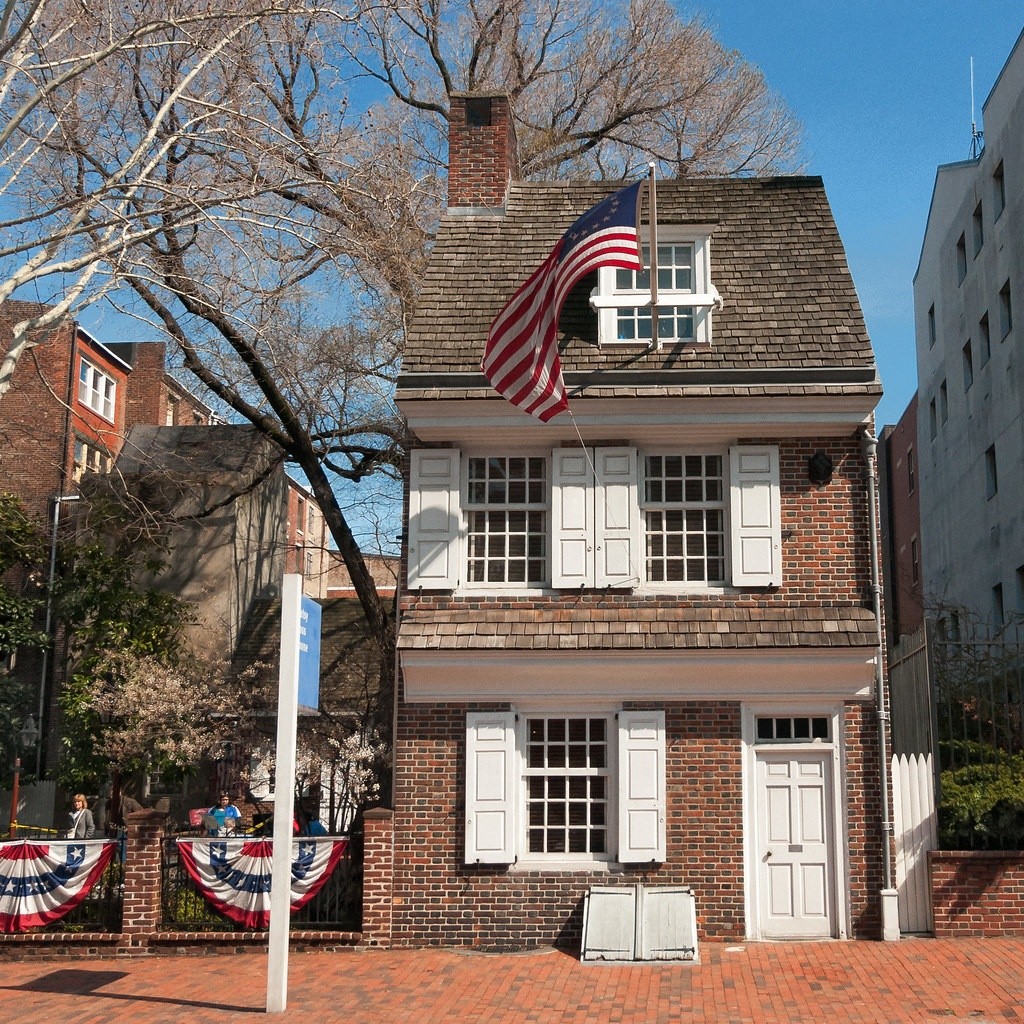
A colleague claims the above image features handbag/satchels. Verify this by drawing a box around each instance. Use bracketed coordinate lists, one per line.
[61, 828, 75, 840]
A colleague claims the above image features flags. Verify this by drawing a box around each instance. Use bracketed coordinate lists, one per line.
[480, 178, 643, 428]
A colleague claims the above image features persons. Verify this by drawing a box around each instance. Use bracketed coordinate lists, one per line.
[303, 808, 327, 836]
[207, 795, 241, 837]
[68, 794, 95, 840]
[104, 786, 144, 839]
